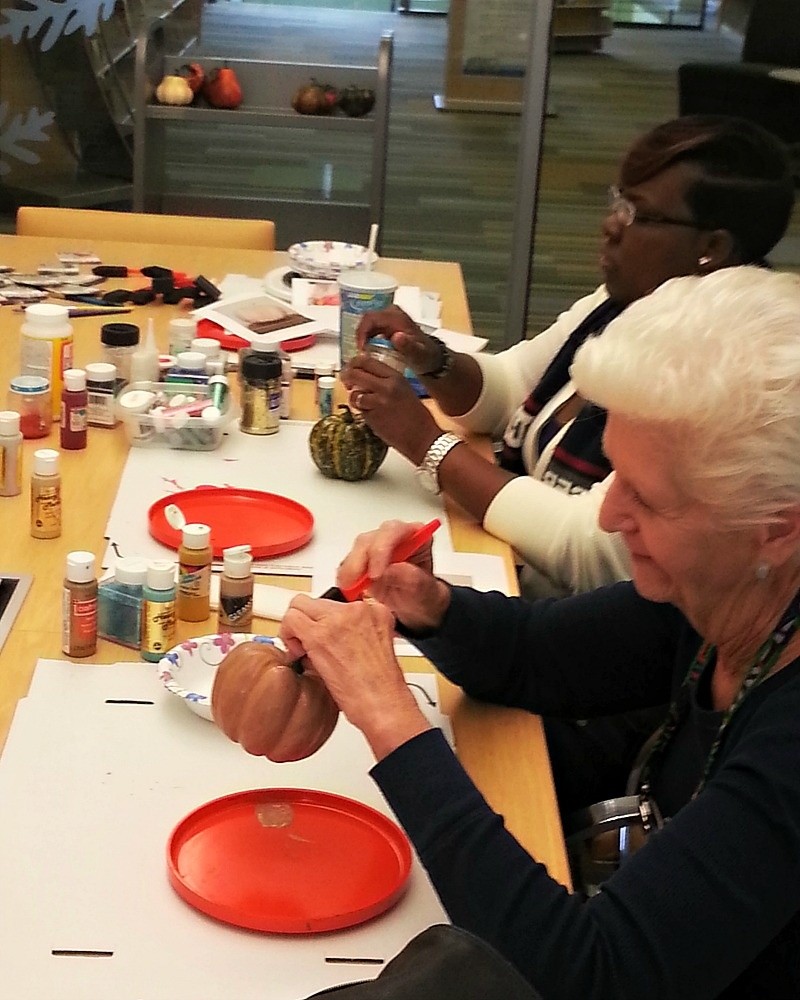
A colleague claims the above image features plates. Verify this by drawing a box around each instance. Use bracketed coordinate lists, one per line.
[288, 240, 379, 269]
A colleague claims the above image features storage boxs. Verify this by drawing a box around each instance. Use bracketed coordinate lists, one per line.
[112, 380, 236, 452]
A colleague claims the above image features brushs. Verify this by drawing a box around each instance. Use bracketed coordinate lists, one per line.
[318, 519, 442, 603]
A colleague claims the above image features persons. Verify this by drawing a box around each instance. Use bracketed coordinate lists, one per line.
[280, 267, 800, 1000]
[343, 117, 795, 601]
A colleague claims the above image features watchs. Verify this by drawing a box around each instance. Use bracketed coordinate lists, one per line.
[415, 432, 465, 495]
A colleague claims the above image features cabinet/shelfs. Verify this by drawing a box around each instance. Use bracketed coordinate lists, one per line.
[20, 0, 202, 181]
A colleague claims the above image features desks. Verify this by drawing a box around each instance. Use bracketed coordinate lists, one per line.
[0, 232, 575, 1000]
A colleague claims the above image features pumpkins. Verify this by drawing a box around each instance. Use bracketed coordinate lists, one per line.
[309, 404, 388, 481]
[156, 60, 242, 108]
[211, 641, 339, 762]
[292, 76, 375, 116]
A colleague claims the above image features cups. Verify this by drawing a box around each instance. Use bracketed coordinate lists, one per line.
[336, 271, 399, 367]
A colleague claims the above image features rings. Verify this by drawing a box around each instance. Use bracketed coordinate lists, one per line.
[355, 393, 364, 409]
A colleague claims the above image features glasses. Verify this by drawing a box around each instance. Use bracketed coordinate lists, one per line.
[608, 185, 715, 229]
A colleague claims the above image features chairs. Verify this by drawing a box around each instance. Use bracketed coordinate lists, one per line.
[16, 207, 275, 250]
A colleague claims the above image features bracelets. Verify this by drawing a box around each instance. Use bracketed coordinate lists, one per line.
[421, 336, 451, 379]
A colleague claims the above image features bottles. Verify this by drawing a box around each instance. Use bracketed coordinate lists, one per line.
[31, 449, 62, 539]
[114, 557, 176, 663]
[219, 544, 254, 626]
[168, 319, 197, 356]
[349, 338, 407, 393]
[0, 411, 23, 496]
[60, 369, 88, 450]
[587, 794, 649, 861]
[6, 376, 51, 438]
[119, 391, 155, 438]
[100, 323, 140, 390]
[314, 365, 335, 418]
[165, 503, 212, 622]
[62, 551, 98, 657]
[86, 364, 124, 429]
[137, 319, 159, 383]
[177, 338, 221, 385]
[153, 375, 229, 446]
[238, 355, 282, 435]
[18, 304, 74, 422]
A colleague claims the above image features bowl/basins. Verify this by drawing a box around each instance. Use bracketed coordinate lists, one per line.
[157, 634, 289, 722]
[115, 382, 235, 451]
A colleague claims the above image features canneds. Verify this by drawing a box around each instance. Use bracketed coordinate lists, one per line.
[83, 322, 142, 430]
[6, 376, 53, 439]
[354, 338, 406, 392]
